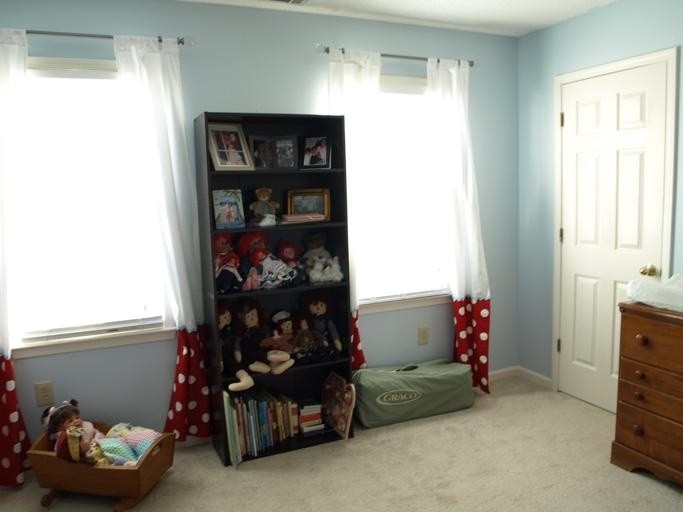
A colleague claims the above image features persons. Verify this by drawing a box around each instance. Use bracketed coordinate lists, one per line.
[39, 401, 162, 468]
[303, 138, 326, 165]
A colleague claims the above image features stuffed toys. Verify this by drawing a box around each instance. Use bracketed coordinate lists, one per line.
[270, 310, 306, 360]
[217, 305, 271, 392]
[276, 237, 310, 285]
[237, 227, 304, 289]
[237, 301, 295, 375]
[249, 186, 281, 225]
[296, 298, 342, 363]
[212, 230, 243, 295]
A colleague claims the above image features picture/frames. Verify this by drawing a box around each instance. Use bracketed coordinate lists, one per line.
[271, 134, 298, 173]
[212, 189, 246, 229]
[300, 133, 332, 170]
[207, 124, 257, 172]
[249, 134, 270, 171]
[287, 189, 331, 221]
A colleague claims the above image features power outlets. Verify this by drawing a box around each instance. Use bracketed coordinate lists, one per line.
[419, 325, 431, 342]
[33, 381, 52, 407]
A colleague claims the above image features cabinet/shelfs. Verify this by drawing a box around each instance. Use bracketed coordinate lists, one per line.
[609, 302, 683, 490]
[193, 110, 356, 468]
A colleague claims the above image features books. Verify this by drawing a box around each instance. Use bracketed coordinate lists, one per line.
[223, 390, 325, 465]
